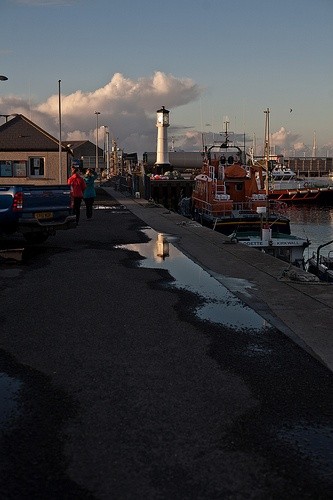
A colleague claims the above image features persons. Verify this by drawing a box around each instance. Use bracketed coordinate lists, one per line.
[67, 168, 85, 222]
[83, 170, 97, 219]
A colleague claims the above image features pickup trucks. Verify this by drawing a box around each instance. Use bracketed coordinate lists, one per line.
[0, 184, 76, 244]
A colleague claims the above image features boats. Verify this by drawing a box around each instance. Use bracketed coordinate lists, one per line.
[182, 107, 333, 281]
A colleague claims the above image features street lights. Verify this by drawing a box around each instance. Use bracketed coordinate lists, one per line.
[58, 80, 62, 184]
[95, 111, 100, 168]
